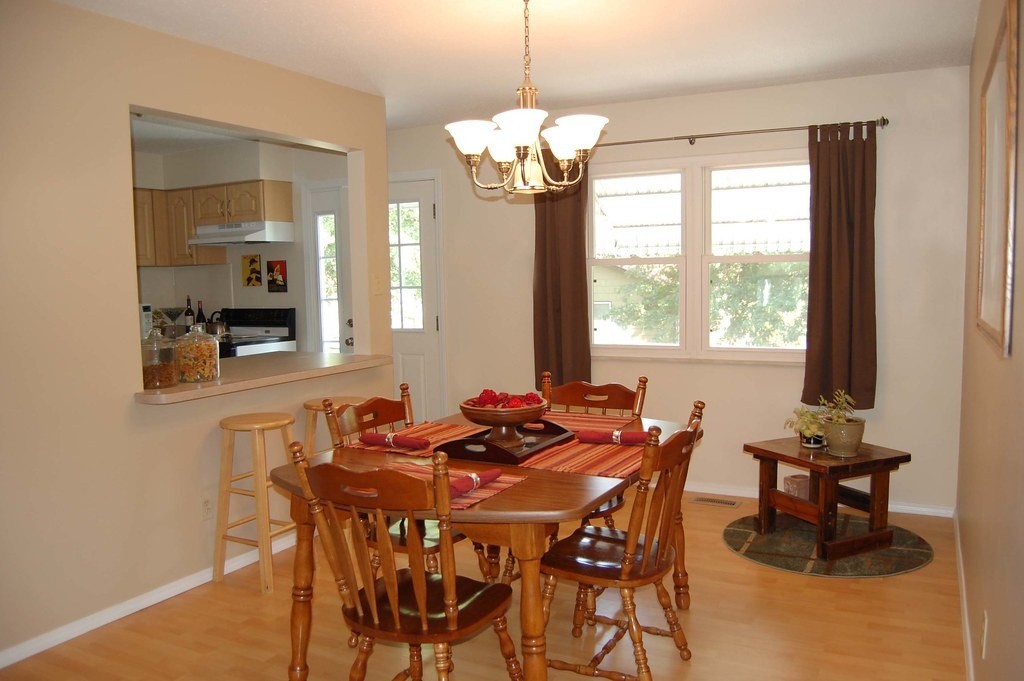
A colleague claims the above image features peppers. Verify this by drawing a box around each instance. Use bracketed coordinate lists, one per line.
[479, 389, 542, 408]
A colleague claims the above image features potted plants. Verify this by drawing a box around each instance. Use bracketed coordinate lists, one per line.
[784, 388, 865, 457]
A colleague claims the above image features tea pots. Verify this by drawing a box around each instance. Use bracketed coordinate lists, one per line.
[205, 311, 225, 334]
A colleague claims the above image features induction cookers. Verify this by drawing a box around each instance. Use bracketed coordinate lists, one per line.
[219, 307, 296, 357]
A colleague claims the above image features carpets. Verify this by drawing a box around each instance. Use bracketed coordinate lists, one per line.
[721, 510, 934, 578]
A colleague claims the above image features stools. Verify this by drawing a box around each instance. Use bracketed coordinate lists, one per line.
[213, 411, 296, 589]
[304, 396, 370, 457]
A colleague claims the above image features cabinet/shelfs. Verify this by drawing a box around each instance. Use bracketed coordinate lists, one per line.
[133, 178, 292, 268]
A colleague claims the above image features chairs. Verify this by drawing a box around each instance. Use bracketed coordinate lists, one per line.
[499, 371, 648, 587]
[321, 381, 491, 585]
[287, 440, 525, 681]
[539, 399, 706, 681]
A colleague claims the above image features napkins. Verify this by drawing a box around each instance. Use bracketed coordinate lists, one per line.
[576, 429, 649, 445]
[450, 468, 501, 498]
[356, 432, 430, 449]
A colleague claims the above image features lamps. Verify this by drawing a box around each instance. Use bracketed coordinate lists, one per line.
[444, 0, 609, 196]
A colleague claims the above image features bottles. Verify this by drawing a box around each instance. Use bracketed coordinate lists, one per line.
[175, 325, 221, 382]
[184, 295, 195, 333]
[196, 300, 206, 334]
[141, 327, 179, 389]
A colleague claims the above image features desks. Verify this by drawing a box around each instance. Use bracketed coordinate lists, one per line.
[269, 415, 703, 681]
[743, 436, 911, 559]
